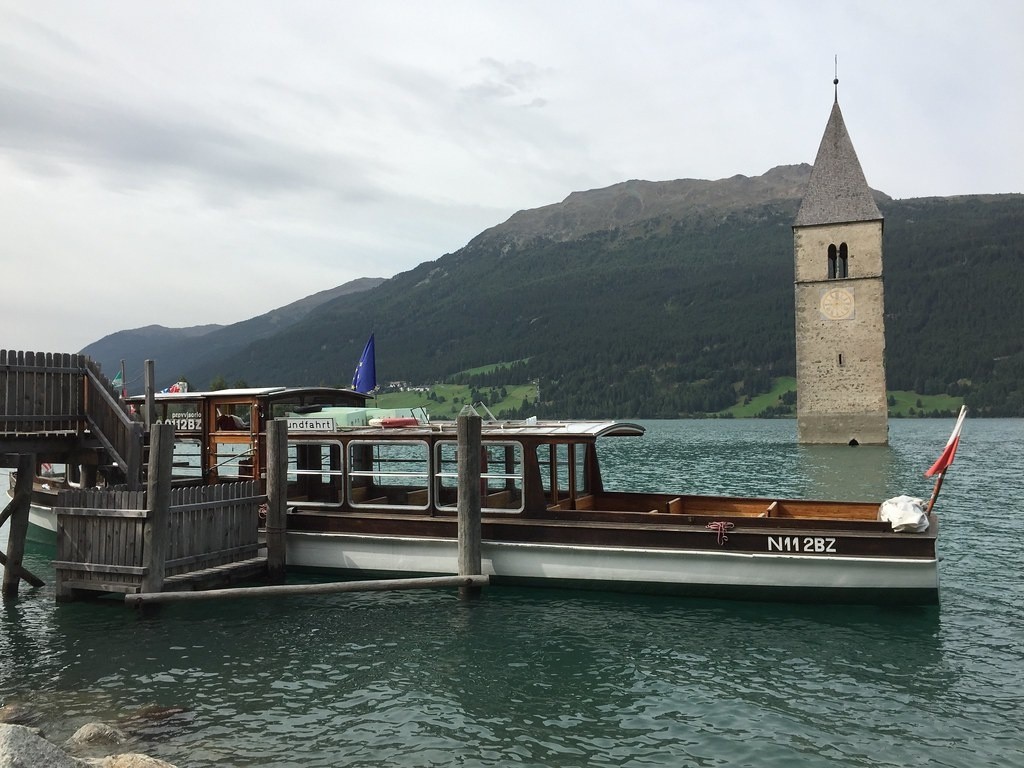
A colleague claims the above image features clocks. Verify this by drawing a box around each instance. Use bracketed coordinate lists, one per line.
[818, 287, 855, 322]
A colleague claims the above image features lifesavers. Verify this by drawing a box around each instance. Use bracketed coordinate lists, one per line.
[368, 417, 420, 429]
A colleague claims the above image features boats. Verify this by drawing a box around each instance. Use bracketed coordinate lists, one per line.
[5, 385, 938, 595]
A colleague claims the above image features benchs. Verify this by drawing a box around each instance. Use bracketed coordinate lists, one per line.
[649, 498, 779, 517]
[288, 488, 522, 511]
